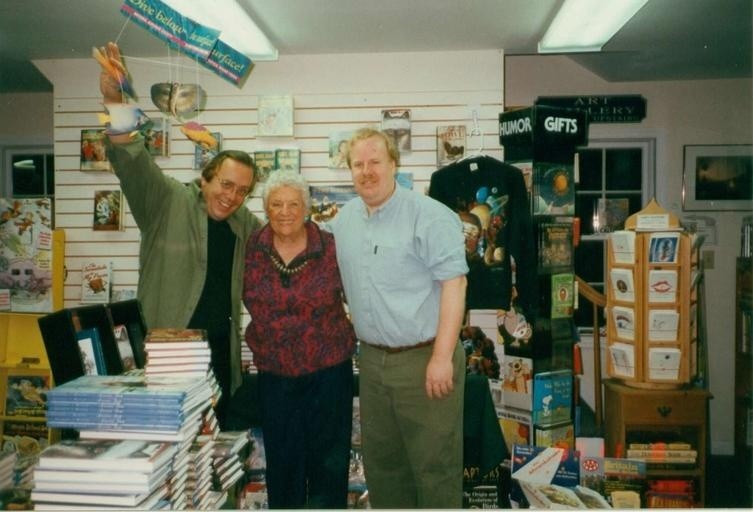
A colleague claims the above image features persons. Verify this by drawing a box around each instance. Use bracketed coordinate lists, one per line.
[100, 40, 268, 432]
[319, 128, 470, 509]
[332, 139, 348, 168]
[239, 167, 355, 509]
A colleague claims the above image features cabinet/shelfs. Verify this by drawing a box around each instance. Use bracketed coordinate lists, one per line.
[0, 311, 61, 510]
[599, 378, 714, 505]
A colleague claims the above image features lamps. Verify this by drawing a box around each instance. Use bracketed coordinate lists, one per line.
[160, 0, 279, 62]
[537, 0, 648, 54]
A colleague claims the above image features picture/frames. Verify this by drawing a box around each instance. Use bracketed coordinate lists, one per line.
[681, 144, 753, 212]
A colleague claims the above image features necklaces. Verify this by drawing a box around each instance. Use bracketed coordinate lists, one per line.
[269, 254, 308, 275]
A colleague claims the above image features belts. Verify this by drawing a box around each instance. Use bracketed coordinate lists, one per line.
[361, 342, 433, 353]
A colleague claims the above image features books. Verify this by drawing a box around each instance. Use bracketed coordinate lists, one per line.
[79, 261, 110, 305]
[109, 288, 137, 305]
[164, 122, 172, 159]
[742, 310, 753, 353]
[144, 117, 165, 156]
[487, 160, 698, 508]
[92, 189, 124, 232]
[275, 148, 300, 175]
[737, 215, 753, 257]
[326, 130, 352, 170]
[745, 408, 752, 446]
[252, 150, 275, 181]
[80, 127, 112, 171]
[380, 109, 411, 153]
[436, 125, 467, 168]
[0, 326, 374, 508]
[394, 171, 412, 191]
[308, 185, 359, 234]
[194, 132, 222, 170]
[256, 95, 295, 139]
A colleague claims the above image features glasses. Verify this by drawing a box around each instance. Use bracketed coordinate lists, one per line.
[214, 172, 248, 198]
